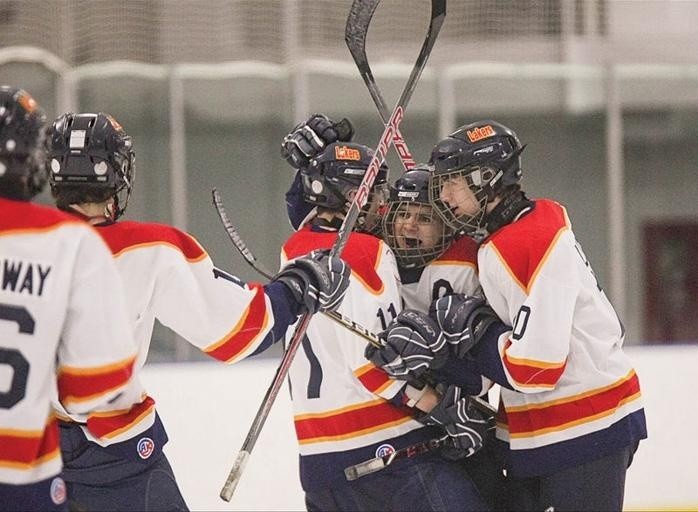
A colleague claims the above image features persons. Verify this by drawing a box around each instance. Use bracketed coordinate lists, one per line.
[42, 108, 352, 511]
[0, 84, 138, 512]
[427, 119, 648, 512]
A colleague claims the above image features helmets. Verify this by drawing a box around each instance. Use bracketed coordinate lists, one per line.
[428, 119, 521, 237]
[0, 84, 50, 199]
[381, 166, 453, 272]
[302, 141, 391, 237]
[46, 111, 136, 220]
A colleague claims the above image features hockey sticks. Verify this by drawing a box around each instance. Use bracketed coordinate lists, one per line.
[220, 2, 449, 503]
[345, 0, 414, 170]
[210, 186, 495, 418]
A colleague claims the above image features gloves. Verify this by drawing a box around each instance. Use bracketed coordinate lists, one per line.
[365, 309, 446, 382]
[429, 292, 499, 372]
[280, 113, 355, 168]
[416, 383, 488, 461]
[280, 248, 350, 313]
